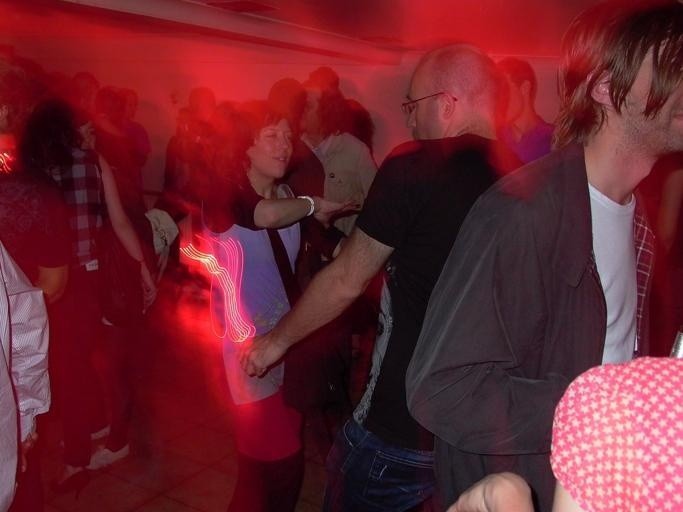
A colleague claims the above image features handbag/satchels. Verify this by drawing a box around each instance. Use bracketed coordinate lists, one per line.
[282, 235, 358, 419]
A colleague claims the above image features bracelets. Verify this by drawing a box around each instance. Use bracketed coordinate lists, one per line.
[140, 258, 145, 264]
[298, 196, 314, 216]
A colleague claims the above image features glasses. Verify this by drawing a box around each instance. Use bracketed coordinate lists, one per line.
[399, 91, 457, 117]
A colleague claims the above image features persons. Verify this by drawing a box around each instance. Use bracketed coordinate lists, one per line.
[234, 43, 518, 512]
[440, 356, 683, 512]
[2, 70, 159, 508]
[149, 67, 378, 509]
[234, 39, 518, 512]
[401, 0, 682, 512]
[497, 52, 556, 165]
